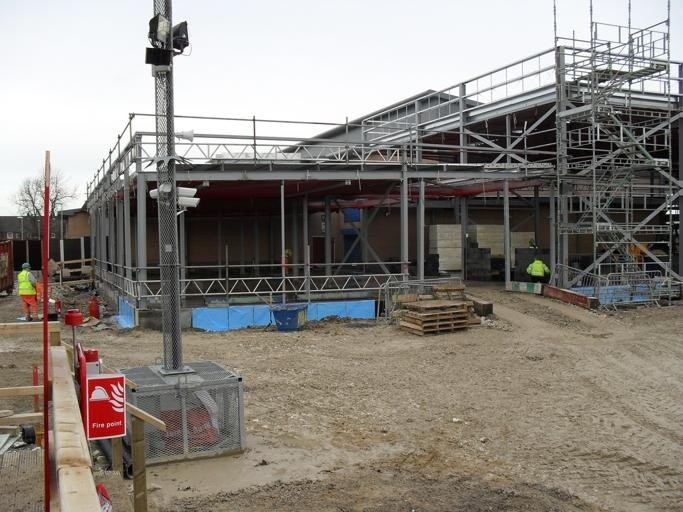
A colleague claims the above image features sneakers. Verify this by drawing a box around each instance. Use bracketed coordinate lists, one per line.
[26, 316, 40, 321]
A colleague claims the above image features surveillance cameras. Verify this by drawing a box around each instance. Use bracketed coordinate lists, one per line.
[159, 182, 172, 193]
[149, 189, 159, 199]
[178, 197, 200, 208]
[177, 187, 197, 197]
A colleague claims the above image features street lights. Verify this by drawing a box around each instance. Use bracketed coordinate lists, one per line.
[144, 0, 191, 378]
[35, 219, 40, 241]
[15, 216, 24, 241]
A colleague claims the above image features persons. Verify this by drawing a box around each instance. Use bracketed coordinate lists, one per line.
[527, 256, 550, 283]
[17, 263, 42, 322]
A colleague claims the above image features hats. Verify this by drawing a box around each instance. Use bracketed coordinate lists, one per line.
[21, 263, 32, 268]
